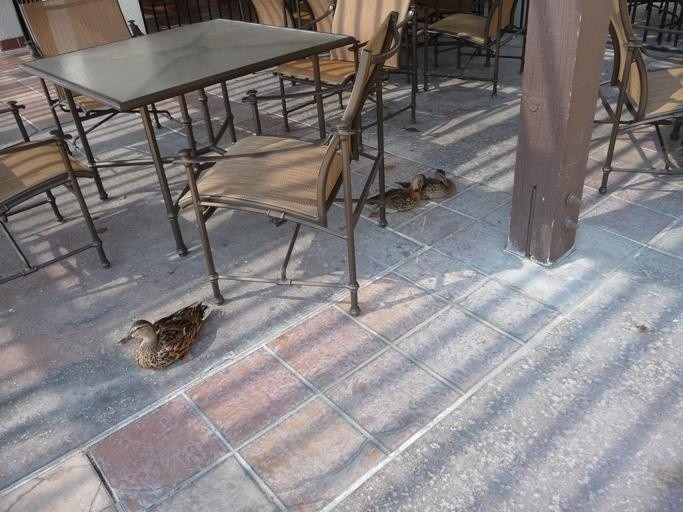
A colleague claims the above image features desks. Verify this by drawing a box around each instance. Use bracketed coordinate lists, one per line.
[19, 19, 358, 256]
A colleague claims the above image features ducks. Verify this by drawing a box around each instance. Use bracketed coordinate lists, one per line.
[118, 300, 209, 371]
[394, 169, 457, 200]
[363, 174, 427, 214]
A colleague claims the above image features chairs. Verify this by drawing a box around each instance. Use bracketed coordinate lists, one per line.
[424, 0, 526, 96]
[2, 1, 162, 285]
[589, 0, 682, 194]
[170, 10, 404, 318]
[255, 2, 416, 151]
